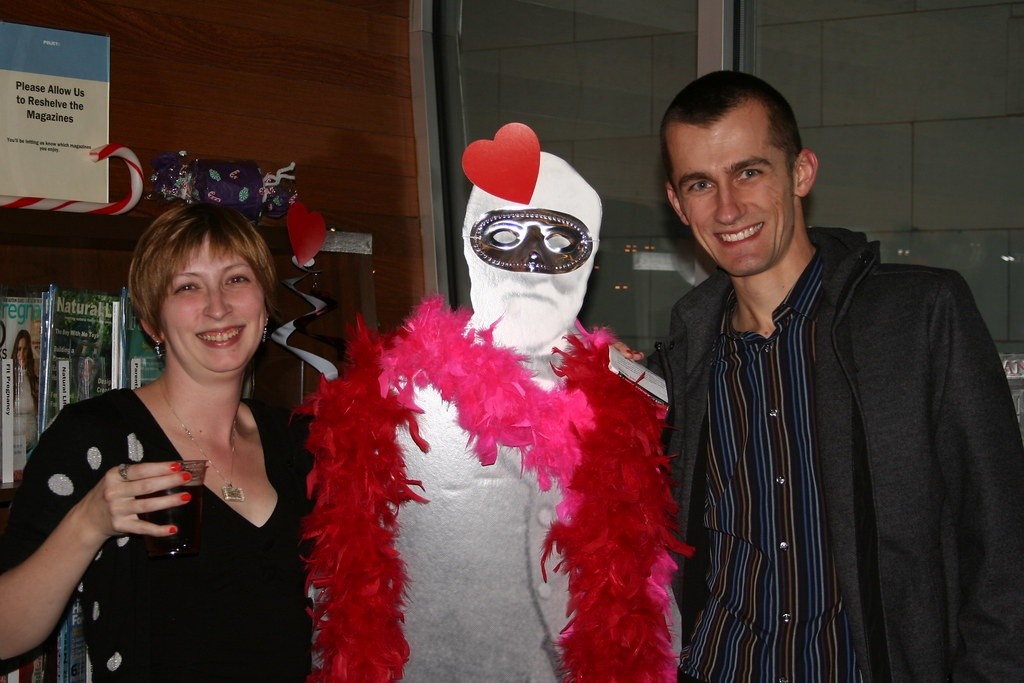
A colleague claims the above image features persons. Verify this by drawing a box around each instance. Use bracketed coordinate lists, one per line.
[0, 201, 647, 682]
[299, 120, 686, 683]
[10, 330, 38, 452]
[646, 67, 1024, 683]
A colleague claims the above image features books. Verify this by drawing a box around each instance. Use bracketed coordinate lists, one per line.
[0, 280, 165, 683]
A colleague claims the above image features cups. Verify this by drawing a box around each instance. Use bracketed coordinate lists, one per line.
[138, 460, 208, 557]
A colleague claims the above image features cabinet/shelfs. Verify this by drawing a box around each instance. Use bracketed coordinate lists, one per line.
[1, 205, 373, 507]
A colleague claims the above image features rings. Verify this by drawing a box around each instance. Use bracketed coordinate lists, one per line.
[119, 461, 130, 479]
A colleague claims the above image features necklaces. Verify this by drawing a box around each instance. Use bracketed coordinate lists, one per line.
[309, 302, 679, 682]
[157, 373, 246, 502]
